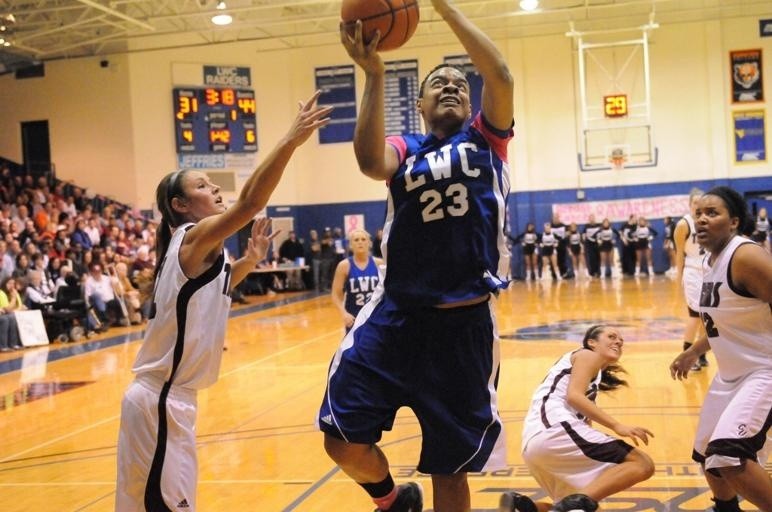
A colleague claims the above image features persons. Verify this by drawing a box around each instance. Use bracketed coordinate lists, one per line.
[665, 184, 771, 511]
[497, 321, 657, 512]
[315, 2, 518, 512]
[115, 82, 334, 512]
[1, 164, 160, 353]
[228, 227, 346, 303]
[671, 187, 710, 372]
[372, 230, 386, 257]
[332, 228, 386, 332]
[750, 207, 771, 252]
[507, 200, 677, 292]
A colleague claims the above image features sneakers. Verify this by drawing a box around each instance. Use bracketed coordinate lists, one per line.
[374, 482, 424, 512]
[500, 491, 598, 512]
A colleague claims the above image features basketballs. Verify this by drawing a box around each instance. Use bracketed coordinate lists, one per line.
[341, 1, 419, 51]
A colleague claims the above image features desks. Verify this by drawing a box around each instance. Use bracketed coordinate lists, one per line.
[240, 262, 312, 294]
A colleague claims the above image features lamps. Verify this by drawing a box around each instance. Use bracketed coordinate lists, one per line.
[211, 1, 234, 26]
